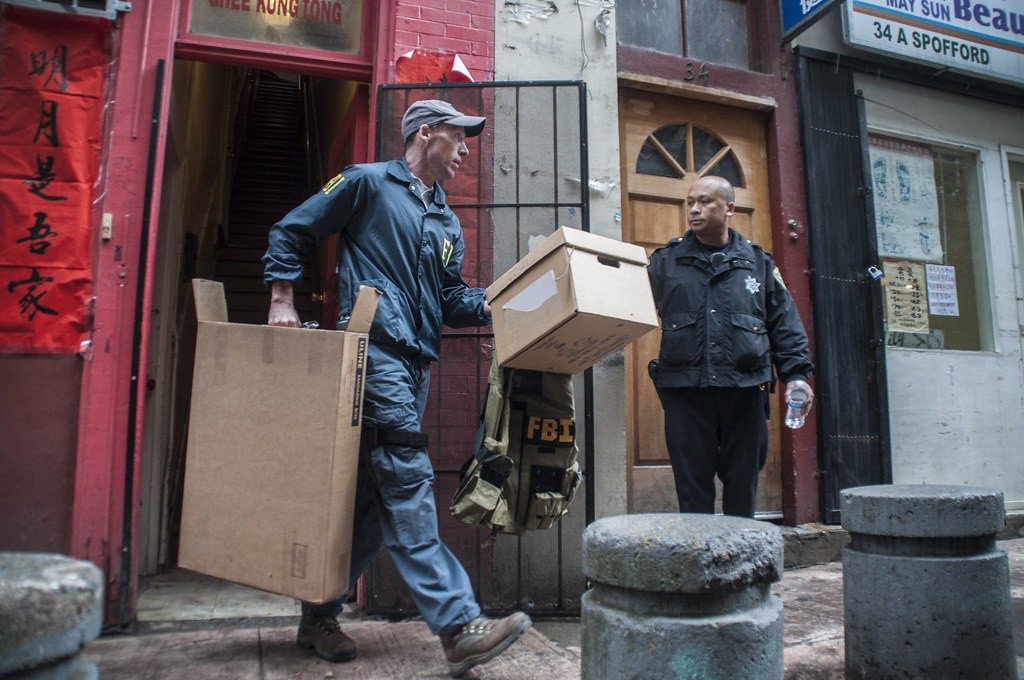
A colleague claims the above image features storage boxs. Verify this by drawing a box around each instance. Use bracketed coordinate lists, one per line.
[485, 226, 659, 375]
[178, 278, 383, 604]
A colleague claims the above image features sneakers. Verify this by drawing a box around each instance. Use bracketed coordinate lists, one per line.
[296, 610, 356, 661]
[440, 611, 532, 677]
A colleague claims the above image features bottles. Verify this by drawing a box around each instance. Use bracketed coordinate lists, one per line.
[785, 376, 811, 429]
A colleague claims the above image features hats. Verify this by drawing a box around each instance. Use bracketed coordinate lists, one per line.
[401, 100, 486, 143]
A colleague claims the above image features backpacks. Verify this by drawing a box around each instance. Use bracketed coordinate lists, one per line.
[447, 339, 582, 539]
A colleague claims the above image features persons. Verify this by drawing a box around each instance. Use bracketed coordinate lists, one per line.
[263, 99, 532, 678]
[648, 176, 814, 519]
